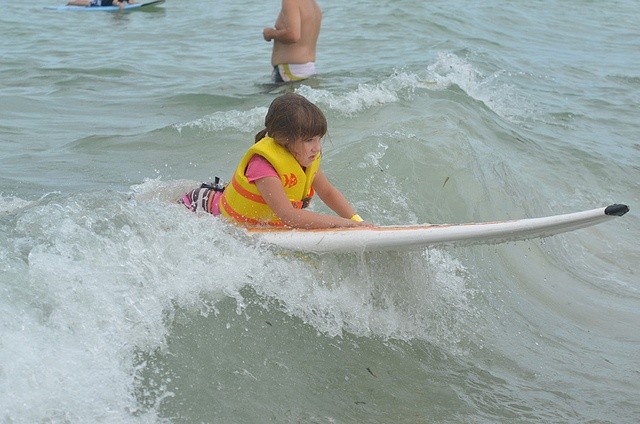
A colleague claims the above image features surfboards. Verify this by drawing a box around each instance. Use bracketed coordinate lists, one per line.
[239, 204, 630, 256]
[44, 0, 165, 12]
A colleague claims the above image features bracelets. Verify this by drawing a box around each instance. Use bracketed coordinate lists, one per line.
[350, 214, 363, 223]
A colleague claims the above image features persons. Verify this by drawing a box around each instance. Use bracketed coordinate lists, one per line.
[65, 0, 136, 14]
[177, 94, 375, 233]
[262, 0, 322, 82]
